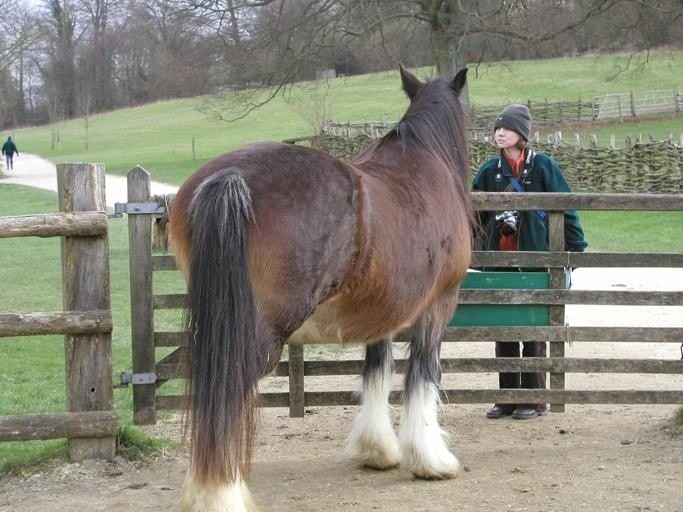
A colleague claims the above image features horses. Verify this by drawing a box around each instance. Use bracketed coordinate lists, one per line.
[166, 59, 488, 512]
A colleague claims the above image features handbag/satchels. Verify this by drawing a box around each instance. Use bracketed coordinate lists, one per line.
[564, 266, 571, 289]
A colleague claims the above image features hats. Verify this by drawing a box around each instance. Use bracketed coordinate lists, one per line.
[494, 104, 531, 142]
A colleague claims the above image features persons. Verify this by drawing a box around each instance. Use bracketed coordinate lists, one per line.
[461, 104, 586, 419]
[2, 136, 19, 170]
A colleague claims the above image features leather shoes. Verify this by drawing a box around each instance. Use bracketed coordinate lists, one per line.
[487, 406, 505, 417]
[512, 408, 546, 419]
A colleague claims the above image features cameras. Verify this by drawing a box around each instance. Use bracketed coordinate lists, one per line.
[493, 211, 523, 236]
